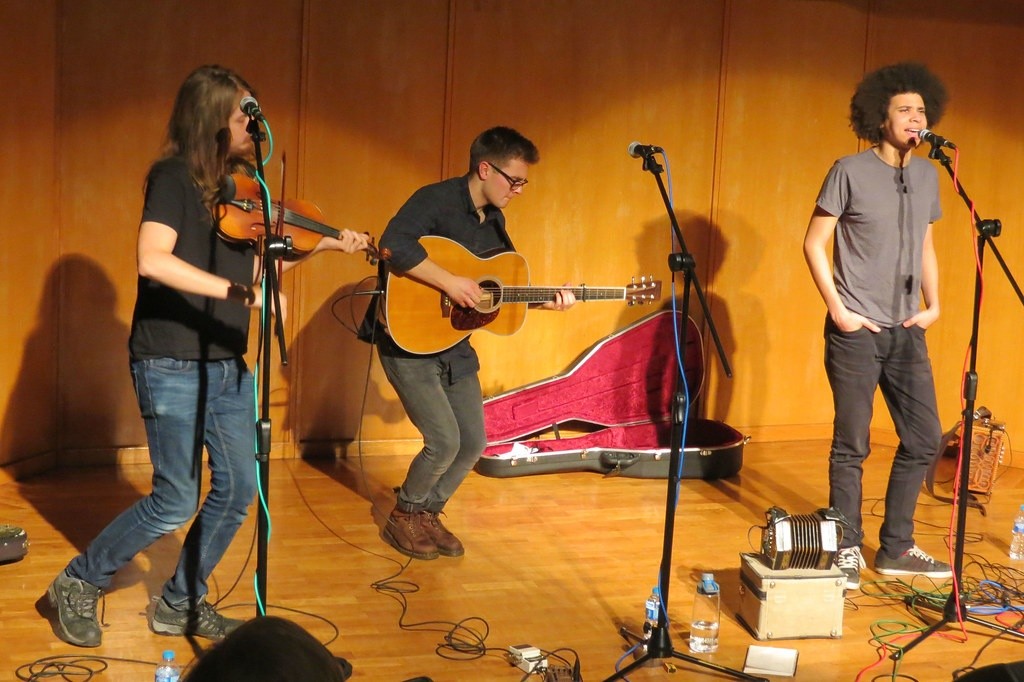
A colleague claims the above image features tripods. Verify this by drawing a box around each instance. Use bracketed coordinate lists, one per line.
[602, 154, 770, 682]
[893, 148, 1024, 660]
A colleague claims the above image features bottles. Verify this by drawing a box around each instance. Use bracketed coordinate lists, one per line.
[155, 651, 180, 682]
[690, 574, 720, 653]
[1009, 506, 1024, 560]
[643, 587, 661, 651]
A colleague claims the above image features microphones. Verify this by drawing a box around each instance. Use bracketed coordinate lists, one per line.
[239, 97, 265, 122]
[917, 129, 957, 150]
[627, 141, 663, 158]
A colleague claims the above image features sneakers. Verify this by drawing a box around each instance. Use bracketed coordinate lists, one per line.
[151, 595, 246, 639]
[383, 508, 438, 559]
[419, 510, 464, 557]
[47, 568, 110, 647]
[835, 546, 866, 589]
[874, 545, 954, 578]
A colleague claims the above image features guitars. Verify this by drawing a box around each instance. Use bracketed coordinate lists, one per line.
[376, 233, 662, 356]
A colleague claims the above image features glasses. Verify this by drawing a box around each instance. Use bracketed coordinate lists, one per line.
[487, 162, 529, 190]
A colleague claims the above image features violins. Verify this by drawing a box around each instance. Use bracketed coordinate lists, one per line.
[213, 170, 394, 265]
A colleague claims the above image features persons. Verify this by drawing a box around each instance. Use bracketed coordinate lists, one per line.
[358, 126, 576, 560]
[803, 61, 953, 589]
[47, 65, 370, 647]
